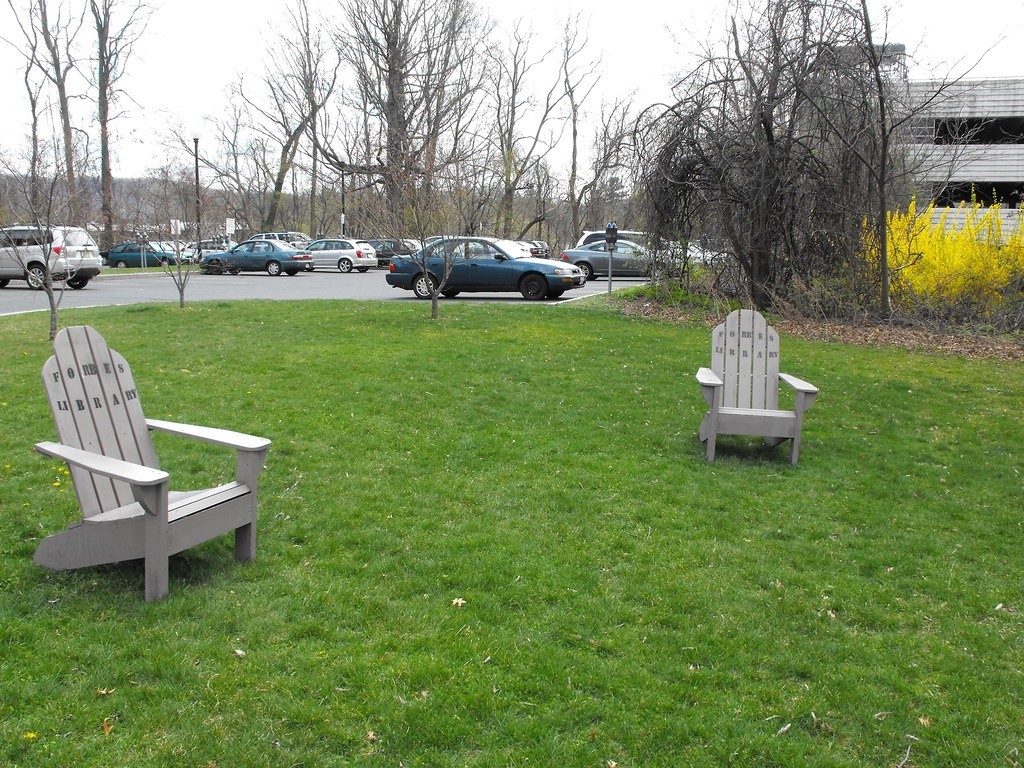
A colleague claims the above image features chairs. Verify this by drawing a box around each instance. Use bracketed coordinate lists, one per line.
[696, 308, 819, 467]
[472, 248, 485, 259]
[328, 244, 333, 249]
[483, 246, 493, 259]
[32, 324, 272, 606]
[334, 244, 339, 249]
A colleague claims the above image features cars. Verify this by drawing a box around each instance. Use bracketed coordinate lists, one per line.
[385, 235, 588, 301]
[198, 239, 316, 276]
[108, 241, 176, 269]
[365, 235, 551, 270]
[560, 239, 685, 281]
[247, 232, 327, 251]
[300, 238, 380, 273]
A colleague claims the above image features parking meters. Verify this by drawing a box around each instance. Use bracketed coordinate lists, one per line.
[604, 222, 618, 295]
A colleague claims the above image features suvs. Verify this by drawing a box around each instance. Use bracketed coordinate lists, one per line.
[573, 228, 689, 260]
[179, 238, 243, 264]
[0, 224, 104, 291]
